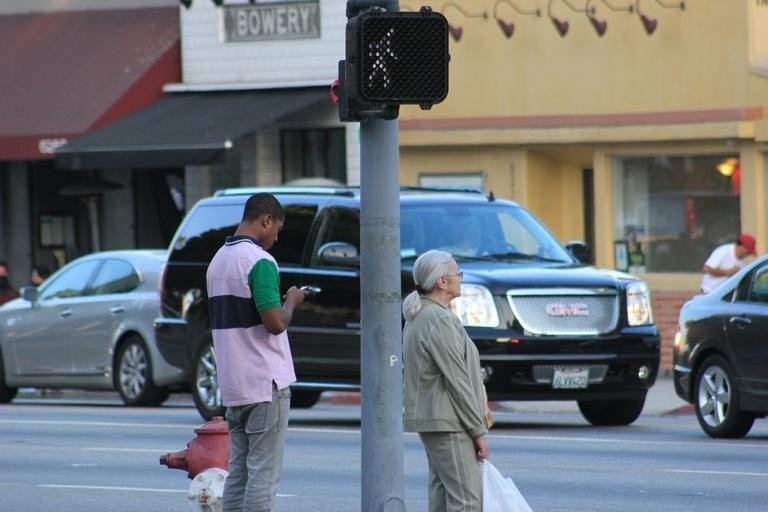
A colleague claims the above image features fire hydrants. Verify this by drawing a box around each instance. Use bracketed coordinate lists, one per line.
[160, 417, 230, 512]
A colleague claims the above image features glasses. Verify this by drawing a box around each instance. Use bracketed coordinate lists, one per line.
[444, 272, 464, 280]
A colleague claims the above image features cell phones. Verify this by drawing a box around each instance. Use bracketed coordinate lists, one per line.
[300, 285, 321, 293]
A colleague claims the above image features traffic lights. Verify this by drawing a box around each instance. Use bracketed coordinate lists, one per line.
[346, 5, 448, 104]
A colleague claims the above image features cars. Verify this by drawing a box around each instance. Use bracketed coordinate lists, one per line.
[0, 248, 190, 406]
[672, 254, 768, 439]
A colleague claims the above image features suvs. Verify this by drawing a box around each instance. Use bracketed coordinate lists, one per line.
[154, 186, 659, 426]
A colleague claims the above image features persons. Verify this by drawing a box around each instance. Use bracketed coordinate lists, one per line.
[699, 234, 757, 296]
[0, 264, 20, 306]
[204, 193, 310, 512]
[32, 261, 59, 287]
[403, 250, 489, 511]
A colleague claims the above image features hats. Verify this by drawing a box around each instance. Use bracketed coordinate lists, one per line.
[738, 235, 757, 256]
